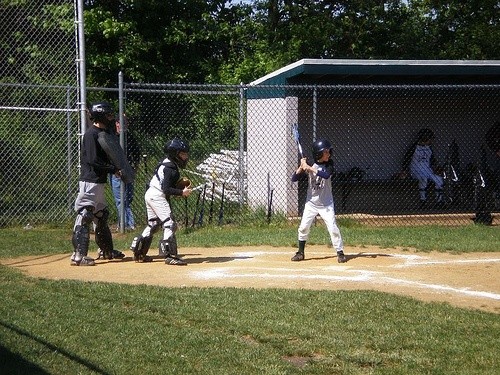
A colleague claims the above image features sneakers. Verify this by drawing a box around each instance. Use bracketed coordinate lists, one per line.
[97, 248, 120, 259]
[71, 251, 95, 266]
[135, 256, 152, 262]
[291, 252, 304, 261]
[165, 254, 186, 265]
[337, 251, 346, 263]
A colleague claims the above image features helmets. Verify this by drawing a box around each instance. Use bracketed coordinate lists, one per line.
[311, 140, 333, 161]
[91, 103, 116, 135]
[162, 139, 190, 169]
[417, 129, 436, 143]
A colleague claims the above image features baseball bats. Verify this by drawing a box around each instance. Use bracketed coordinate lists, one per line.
[190, 189, 201, 228]
[208, 182, 215, 224]
[291, 123, 307, 173]
[266, 190, 273, 225]
[216, 183, 225, 224]
[184, 196, 188, 233]
[199, 185, 208, 225]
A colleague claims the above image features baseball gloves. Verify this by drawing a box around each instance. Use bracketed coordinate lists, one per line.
[176, 177, 191, 199]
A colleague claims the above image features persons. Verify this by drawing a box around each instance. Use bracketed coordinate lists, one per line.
[291, 140, 349, 263]
[399, 128, 444, 205]
[130, 138, 190, 266]
[68, 103, 135, 266]
[108, 116, 135, 232]
